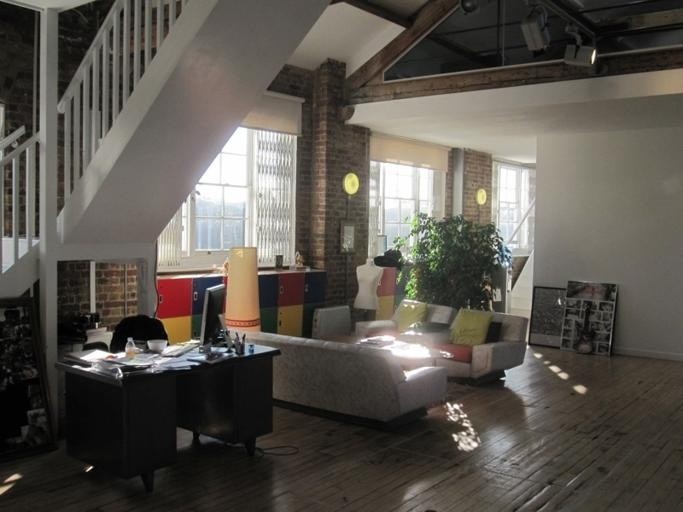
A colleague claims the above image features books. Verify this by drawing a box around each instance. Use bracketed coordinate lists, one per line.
[64, 348, 113, 365]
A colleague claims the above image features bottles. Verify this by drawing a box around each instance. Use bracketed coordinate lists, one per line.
[276, 255, 283, 272]
[126, 337, 136, 361]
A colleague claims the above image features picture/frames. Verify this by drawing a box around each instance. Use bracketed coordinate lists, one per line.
[0, 298, 55, 460]
[528, 285, 566, 348]
[560, 282, 617, 357]
[338, 221, 357, 254]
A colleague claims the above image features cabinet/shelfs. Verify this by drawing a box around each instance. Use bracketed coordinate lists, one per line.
[156, 270, 328, 346]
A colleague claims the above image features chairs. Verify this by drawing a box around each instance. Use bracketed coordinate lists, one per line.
[109, 313, 170, 352]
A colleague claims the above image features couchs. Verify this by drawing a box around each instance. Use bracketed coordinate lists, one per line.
[354, 297, 529, 388]
[242, 332, 448, 431]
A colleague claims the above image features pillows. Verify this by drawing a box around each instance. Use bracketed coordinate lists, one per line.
[449, 308, 494, 347]
[392, 300, 428, 331]
[420, 321, 449, 334]
[485, 319, 501, 343]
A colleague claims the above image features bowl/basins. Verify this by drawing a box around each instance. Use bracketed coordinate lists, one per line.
[146, 338, 168, 352]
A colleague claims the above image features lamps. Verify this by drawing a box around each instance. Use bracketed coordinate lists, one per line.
[342, 170, 359, 195]
[457, 0, 478, 15]
[562, 32, 597, 67]
[476, 185, 488, 205]
[521, 7, 553, 52]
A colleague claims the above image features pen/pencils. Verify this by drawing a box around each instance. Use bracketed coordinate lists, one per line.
[235, 332, 246, 346]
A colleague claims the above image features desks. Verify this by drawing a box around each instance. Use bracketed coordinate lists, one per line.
[56, 336, 282, 494]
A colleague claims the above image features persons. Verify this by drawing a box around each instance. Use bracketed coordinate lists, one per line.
[353, 259, 384, 310]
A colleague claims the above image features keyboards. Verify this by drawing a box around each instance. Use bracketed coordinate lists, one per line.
[162, 343, 199, 357]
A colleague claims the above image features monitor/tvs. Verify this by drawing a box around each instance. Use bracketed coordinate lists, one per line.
[200, 283, 233, 354]
